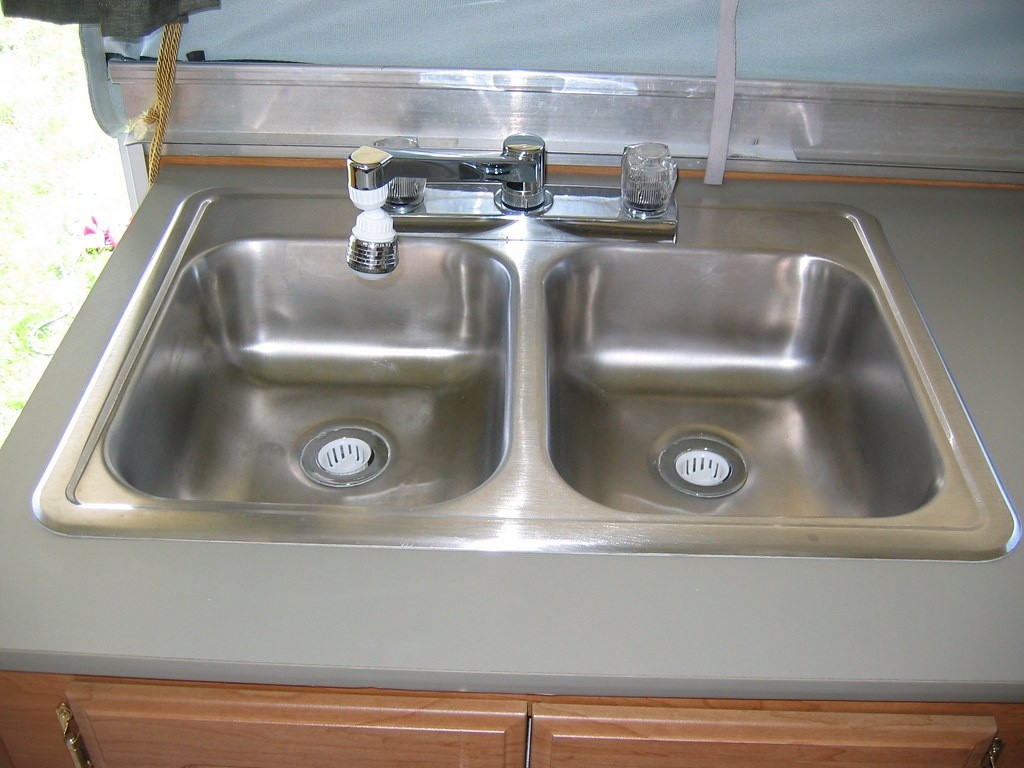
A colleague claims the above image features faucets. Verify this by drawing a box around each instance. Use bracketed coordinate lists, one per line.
[347, 132, 554, 217]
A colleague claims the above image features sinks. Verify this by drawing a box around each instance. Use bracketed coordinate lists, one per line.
[64, 232, 524, 516]
[532, 246, 987, 530]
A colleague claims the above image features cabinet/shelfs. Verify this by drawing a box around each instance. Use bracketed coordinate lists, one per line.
[0, 668, 1024, 768]
